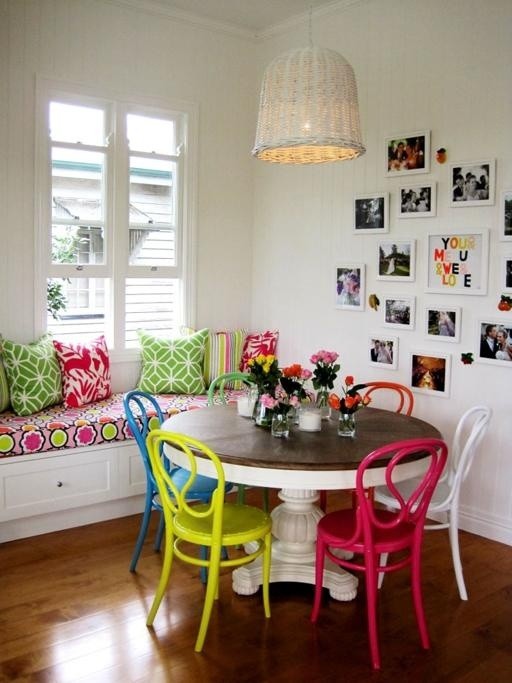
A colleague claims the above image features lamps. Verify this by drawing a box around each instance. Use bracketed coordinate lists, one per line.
[249, 0, 368, 167]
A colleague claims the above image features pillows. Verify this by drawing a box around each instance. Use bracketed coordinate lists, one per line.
[0, 361, 16, 416]
[1, 332, 65, 418]
[133, 325, 213, 398]
[181, 323, 248, 394]
[241, 327, 279, 369]
[53, 333, 115, 409]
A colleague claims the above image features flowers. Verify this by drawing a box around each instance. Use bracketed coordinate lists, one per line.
[245, 348, 341, 414]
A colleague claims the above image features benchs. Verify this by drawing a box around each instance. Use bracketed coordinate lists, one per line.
[0, 390, 260, 545]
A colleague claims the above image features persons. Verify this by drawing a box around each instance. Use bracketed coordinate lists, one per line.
[401, 188, 431, 212]
[356, 199, 383, 228]
[373, 299, 512, 391]
[380, 247, 405, 276]
[454, 166, 490, 201]
[337, 270, 359, 305]
[387, 139, 425, 169]
